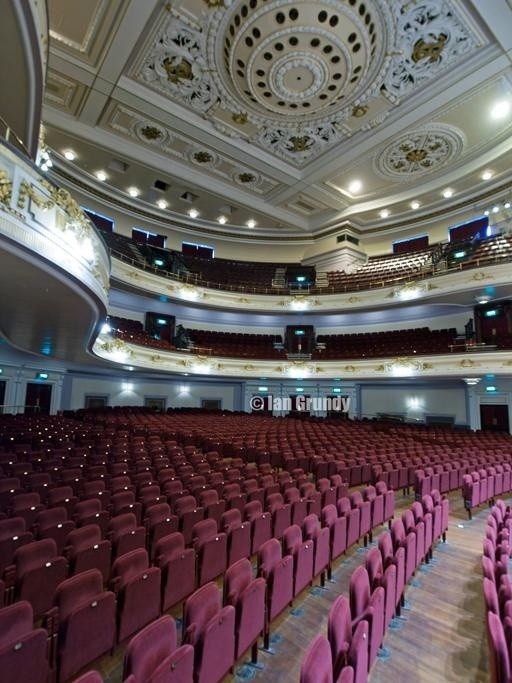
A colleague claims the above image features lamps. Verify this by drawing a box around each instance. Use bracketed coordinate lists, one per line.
[473, 294, 489, 306]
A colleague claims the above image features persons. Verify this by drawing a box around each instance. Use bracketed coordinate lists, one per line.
[176, 324, 186, 348]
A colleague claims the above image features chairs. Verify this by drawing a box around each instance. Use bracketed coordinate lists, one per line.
[108, 313, 458, 360]
[0, 404, 512, 682]
[98, 227, 512, 294]
